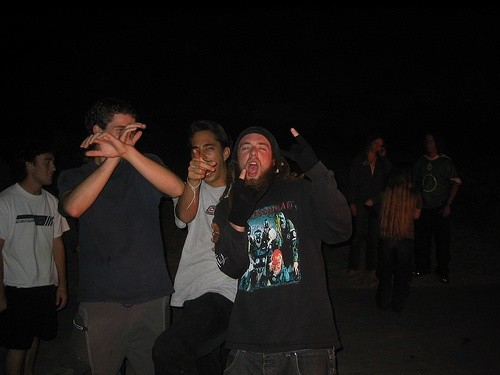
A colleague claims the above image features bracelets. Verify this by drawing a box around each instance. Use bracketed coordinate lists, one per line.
[186, 177, 202, 211]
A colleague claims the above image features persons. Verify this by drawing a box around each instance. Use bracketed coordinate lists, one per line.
[0, 143, 69, 375]
[212, 126, 353, 375]
[405, 127, 458, 286]
[348, 135, 392, 267]
[365, 168, 421, 310]
[152, 120, 238, 375]
[57, 101, 186, 375]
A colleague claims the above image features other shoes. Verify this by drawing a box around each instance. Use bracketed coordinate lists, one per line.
[415, 272, 425, 277]
[393, 302, 405, 310]
[377, 302, 389, 310]
[440, 278, 449, 284]
